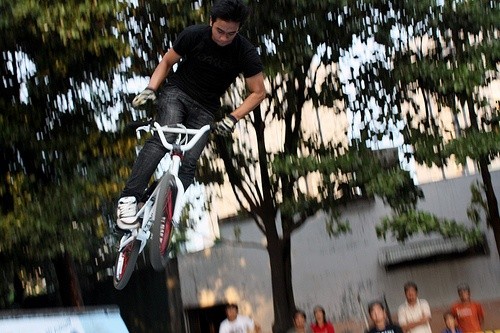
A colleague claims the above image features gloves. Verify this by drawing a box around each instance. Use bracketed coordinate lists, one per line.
[132, 87, 158, 110]
[215, 113, 238, 137]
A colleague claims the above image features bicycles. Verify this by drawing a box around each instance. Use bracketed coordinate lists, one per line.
[113, 121, 234, 290]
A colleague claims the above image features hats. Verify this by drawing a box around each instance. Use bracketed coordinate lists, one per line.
[457, 283, 471, 289]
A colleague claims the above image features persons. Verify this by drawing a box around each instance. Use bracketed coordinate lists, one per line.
[288, 309, 310, 333]
[440, 312, 462, 333]
[449, 283, 484, 333]
[364, 301, 403, 333]
[310, 305, 335, 333]
[117, 0, 267, 229]
[218, 304, 256, 333]
[398, 281, 432, 333]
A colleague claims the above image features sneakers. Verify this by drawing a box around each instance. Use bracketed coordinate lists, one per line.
[117, 196, 140, 229]
